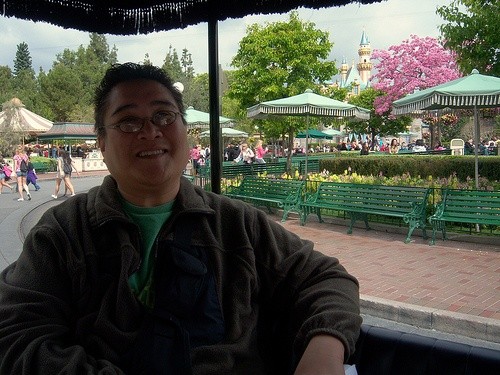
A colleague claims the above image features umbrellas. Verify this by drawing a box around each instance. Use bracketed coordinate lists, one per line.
[198, 127, 248, 163]
[0, 99, 54, 145]
[181, 107, 234, 130]
[246, 89, 372, 200]
[293, 129, 333, 140]
[393, 70, 500, 233]
[320, 127, 347, 137]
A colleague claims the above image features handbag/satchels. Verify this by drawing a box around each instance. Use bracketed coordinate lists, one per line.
[248, 150, 255, 162]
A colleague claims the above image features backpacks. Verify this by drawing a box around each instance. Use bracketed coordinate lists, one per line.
[63, 159, 72, 174]
[19, 155, 28, 172]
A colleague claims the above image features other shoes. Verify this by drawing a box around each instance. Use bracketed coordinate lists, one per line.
[17, 198, 24, 202]
[51, 195, 58, 200]
[27, 193, 32, 201]
[70, 193, 76, 198]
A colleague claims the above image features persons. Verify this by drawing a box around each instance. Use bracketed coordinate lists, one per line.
[274, 143, 306, 158]
[187, 144, 240, 177]
[362, 134, 404, 156]
[27, 148, 49, 158]
[50, 150, 80, 199]
[464, 135, 500, 156]
[407, 137, 446, 154]
[309, 139, 361, 153]
[0, 63, 364, 375]
[0, 151, 14, 195]
[51, 145, 90, 159]
[12, 145, 40, 201]
[233, 140, 268, 172]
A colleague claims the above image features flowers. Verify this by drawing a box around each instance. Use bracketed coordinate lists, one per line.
[422, 111, 458, 126]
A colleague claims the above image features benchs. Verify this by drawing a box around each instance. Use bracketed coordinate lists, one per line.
[30, 162, 49, 174]
[183, 145, 500, 245]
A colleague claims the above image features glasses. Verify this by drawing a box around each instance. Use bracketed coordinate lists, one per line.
[102, 110, 184, 133]
